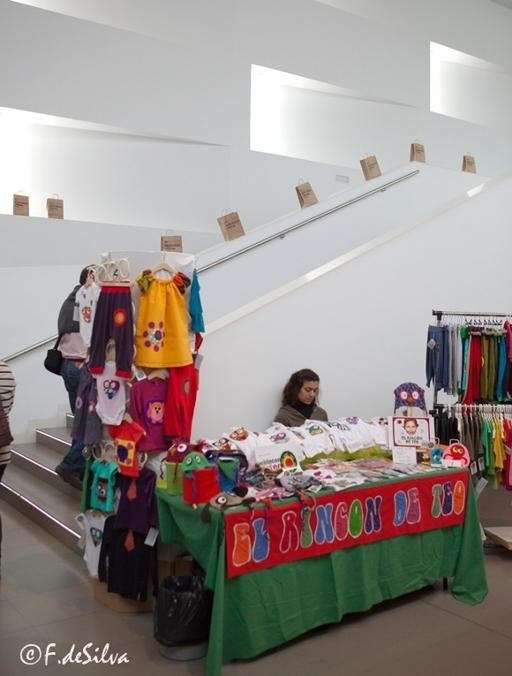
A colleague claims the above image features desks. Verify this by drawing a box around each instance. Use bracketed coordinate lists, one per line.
[153, 458, 473, 662]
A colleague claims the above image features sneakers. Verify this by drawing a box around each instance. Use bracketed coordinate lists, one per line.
[55, 462, 83, 492]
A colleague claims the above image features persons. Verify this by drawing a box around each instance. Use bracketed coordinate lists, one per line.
[403, 418, 418, 435]
[0, 359, 18, 550]
[52, 264, 105, 493]
[273, 367, 328, 428]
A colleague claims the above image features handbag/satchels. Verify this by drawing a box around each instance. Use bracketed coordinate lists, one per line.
[44, 349, 63, 374]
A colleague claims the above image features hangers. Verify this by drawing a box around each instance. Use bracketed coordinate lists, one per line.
[85, 253, 175, 519]
[443, 404, 512, 422]
[435, 311, 512, 329]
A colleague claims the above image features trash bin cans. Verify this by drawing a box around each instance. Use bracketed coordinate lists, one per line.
[154, 577, 213, 660]
